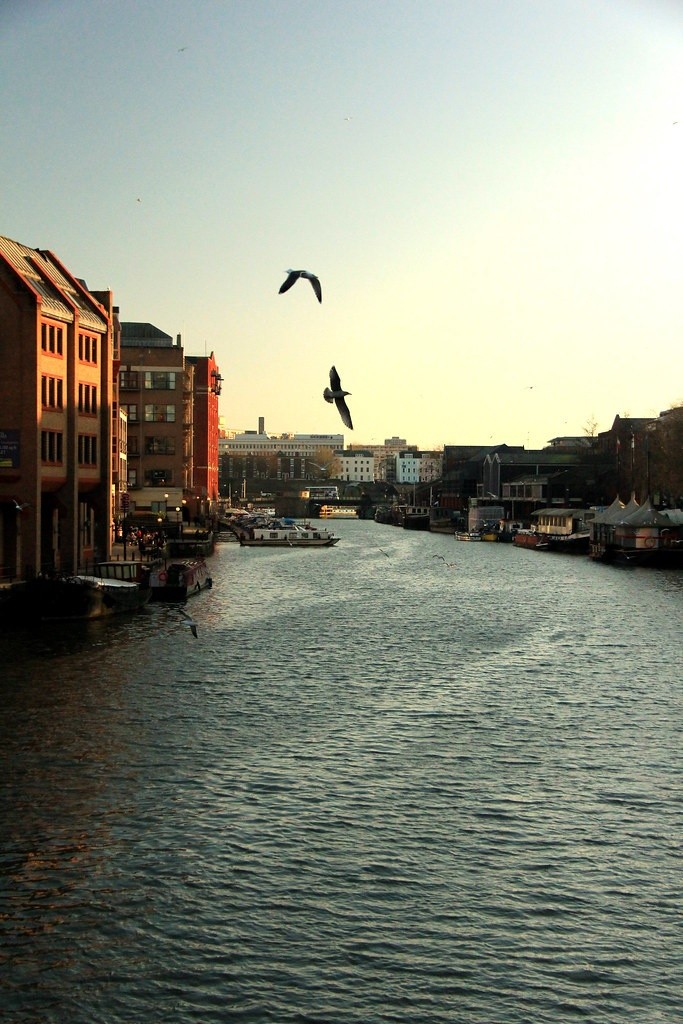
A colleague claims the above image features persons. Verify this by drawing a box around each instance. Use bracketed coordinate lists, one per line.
[126, 525, 159, 547]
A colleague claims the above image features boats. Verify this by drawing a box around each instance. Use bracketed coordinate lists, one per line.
[238, 527, 342, 546]
[152, 557, 216, 603]
[29, 558, 157, 622]
[224, 507, 317, 531]
[453, 530, 482, 542]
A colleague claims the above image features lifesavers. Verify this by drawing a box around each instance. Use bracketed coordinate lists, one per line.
[644, 536, 656, 548]
[159, 573, 167, 581]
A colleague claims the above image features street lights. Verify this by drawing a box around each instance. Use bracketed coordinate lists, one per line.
[175, 507, 180, 522]
[164, 493, 169, 517]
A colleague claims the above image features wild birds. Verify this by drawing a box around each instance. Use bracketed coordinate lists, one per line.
[323, 364, 355, 430]
[279, 268, 323, 304]
[379, 548, 457, 568]
[178, 607, 200, 640]
